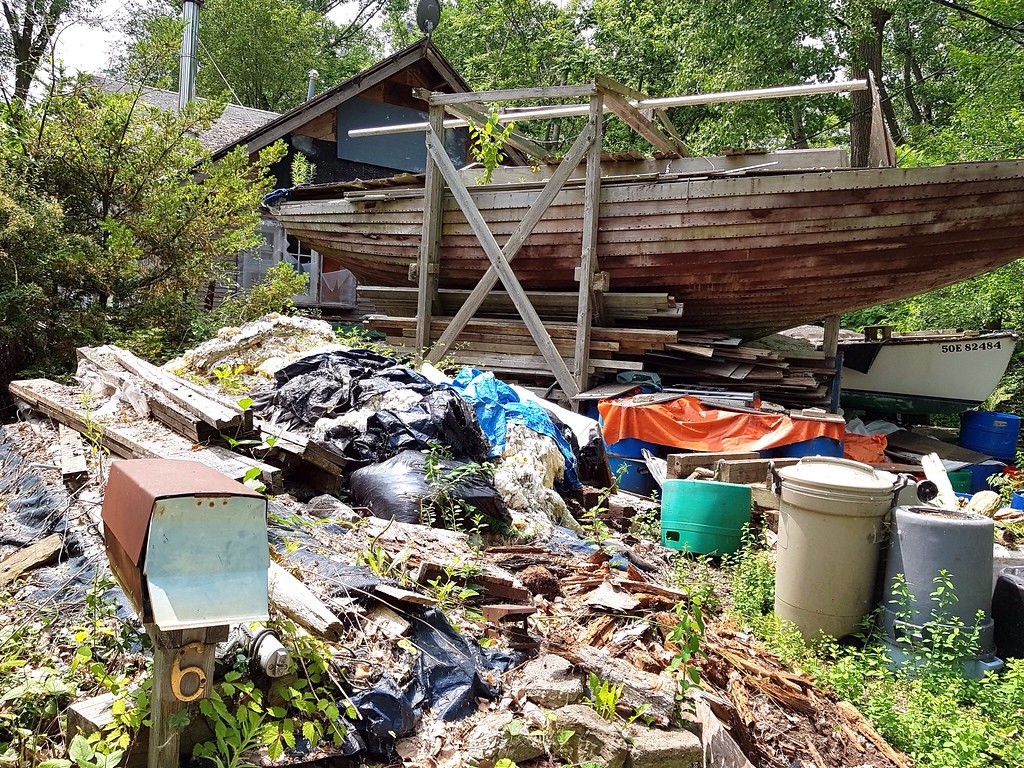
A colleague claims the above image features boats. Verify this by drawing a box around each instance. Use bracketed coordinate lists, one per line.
[813, 316, 1020, 420]
[260, 70, 1024, 351]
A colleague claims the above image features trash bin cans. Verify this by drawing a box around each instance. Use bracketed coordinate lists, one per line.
[775, 456, 895, 648]
[957, 410, 1022, 492]
[874, 506, 1004, 679]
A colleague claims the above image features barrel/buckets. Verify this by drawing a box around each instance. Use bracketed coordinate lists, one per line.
[936, 409, 1024, 513]
[598, 413, 844, 501]
[660, 479, 751, 558]
[767, 456, 894, 654]
[868, 504, 998, 661]
